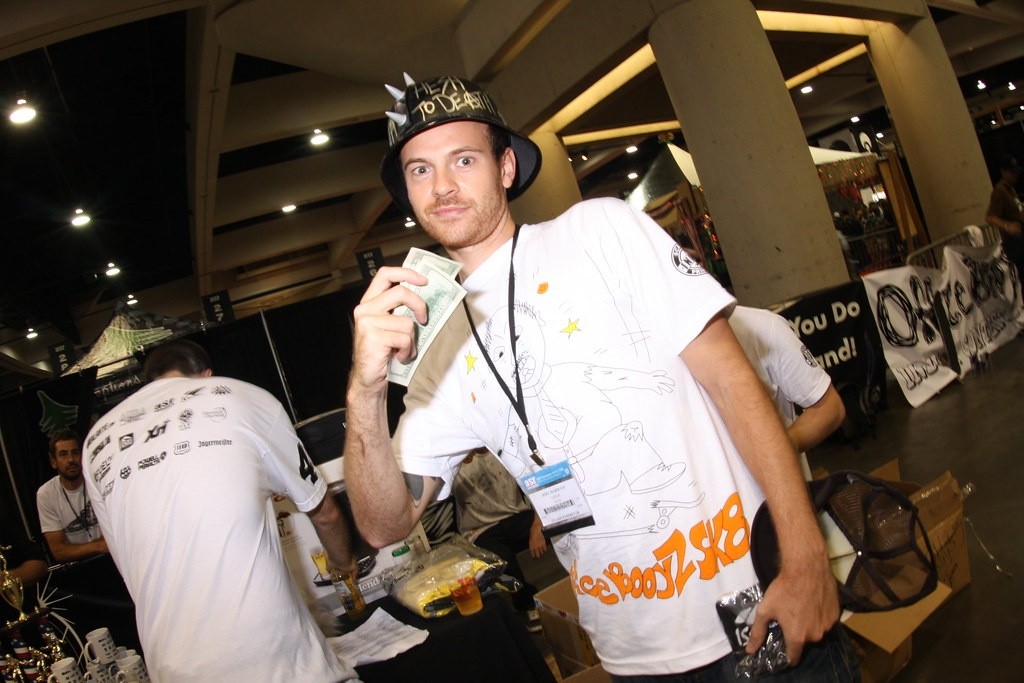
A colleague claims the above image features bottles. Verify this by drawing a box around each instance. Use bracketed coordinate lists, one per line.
[322, 550, 366, 614]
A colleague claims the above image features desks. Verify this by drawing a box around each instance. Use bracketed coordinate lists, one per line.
[0, 554, 144, 672]
[335, 578, 558, 683]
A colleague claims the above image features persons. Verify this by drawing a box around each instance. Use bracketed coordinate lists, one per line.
[684, 247, 856, 587]
[1, 632, 66, 683]
[36, 426, 111, 559]
[984, 158, 1024, 306]
[0, 525, 48, 649]
[81, 341, 363, 683]
[833, 196, 904, 270]
[0, 544, 12, 572]
[453, 446, 548, 631]
[344, 70, 843, 683]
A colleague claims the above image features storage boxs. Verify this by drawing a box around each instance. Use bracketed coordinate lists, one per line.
[532, 576, 600, 676]
[830, 457, 971, 602]
[271, 456, 431, 617]
[838, 561, 953, 683]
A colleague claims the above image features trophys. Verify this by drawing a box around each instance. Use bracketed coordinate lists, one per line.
[0, 567, 30, 621]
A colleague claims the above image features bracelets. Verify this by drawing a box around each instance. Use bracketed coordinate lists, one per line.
[1004, 220, 1009, 232]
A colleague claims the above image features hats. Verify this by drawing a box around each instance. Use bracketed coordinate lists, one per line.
[999, 154, 1023, 172]
[750, 470, 939, 613]
[380, 71, 542, 216]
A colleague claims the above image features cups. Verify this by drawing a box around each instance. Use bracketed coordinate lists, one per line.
[439, 561, 483, 615]
[47, 628, 149, 682]
[310, 545, 328, 576]
